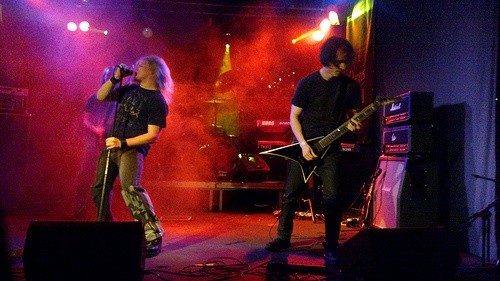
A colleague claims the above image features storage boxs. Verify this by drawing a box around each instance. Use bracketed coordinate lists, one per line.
[383, 90, 433, 156]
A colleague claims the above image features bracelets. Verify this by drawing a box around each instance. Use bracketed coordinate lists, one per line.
[300, 140, 306, 147]
[121, 138, 128, 149]
[110, 76, 120, 84]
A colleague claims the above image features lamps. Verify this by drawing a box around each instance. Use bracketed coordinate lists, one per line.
[224, 34, 232, 48]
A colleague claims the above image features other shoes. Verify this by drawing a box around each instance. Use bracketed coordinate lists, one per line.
[264, 238, 292, 252]
[323, 246, 337, 262]
[145, 236, 162, 258]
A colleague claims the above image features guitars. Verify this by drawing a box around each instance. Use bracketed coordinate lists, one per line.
[257, 96, 403, 184]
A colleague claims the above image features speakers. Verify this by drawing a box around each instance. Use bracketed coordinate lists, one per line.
[21, 220, 145, 281]
[332, 228, 464, 281]
[369, 156, 436, 230]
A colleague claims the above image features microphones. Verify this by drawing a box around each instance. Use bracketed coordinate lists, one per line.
[119, 65, 133, 75]
[373, 168, 381, 181]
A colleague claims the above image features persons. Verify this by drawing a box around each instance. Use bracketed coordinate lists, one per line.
[63, 67, 116, 218]
[264, 35, 368, 265]
[91, 55, 174, 258]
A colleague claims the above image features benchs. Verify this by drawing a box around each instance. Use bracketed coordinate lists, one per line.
[170, 181, 287, 211]
